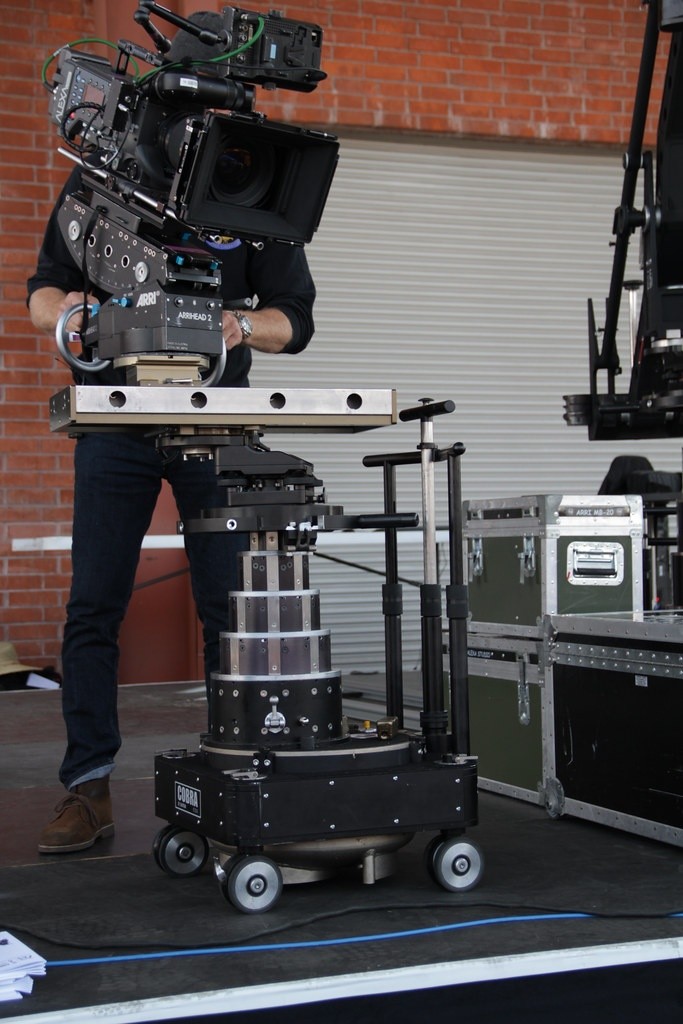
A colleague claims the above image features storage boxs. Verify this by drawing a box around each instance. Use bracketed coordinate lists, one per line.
[458, 492, 683, 850]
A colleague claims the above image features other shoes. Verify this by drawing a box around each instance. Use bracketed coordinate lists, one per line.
[38, 776, 114, 852]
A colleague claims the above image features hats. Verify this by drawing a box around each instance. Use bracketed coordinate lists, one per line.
[165, 12, 229, 75]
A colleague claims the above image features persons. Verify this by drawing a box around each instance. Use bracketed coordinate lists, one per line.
[26, 13, 318, 855]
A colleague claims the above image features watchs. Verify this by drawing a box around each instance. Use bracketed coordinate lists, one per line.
[224, 310, 253, 340]
